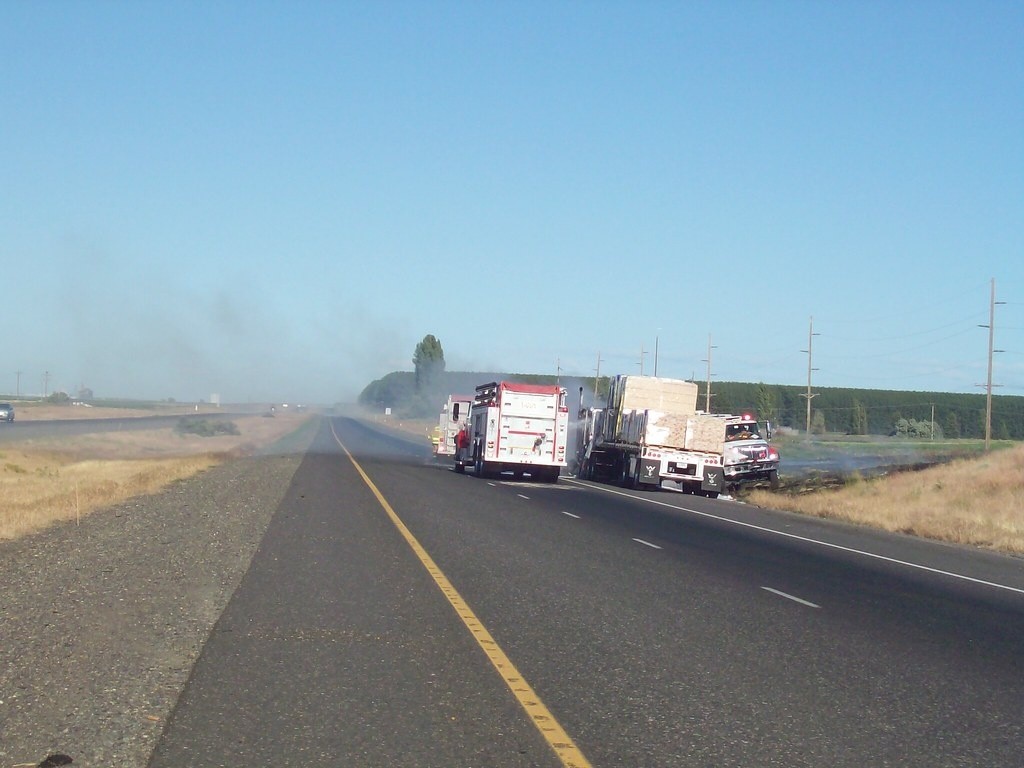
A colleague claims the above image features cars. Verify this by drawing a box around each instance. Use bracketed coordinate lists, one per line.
[0, 401, 15, 422]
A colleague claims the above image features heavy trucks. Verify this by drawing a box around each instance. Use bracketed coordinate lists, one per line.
[573, 374, 727, 498]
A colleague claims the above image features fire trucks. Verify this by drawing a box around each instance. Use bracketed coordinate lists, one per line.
[453, 381, 569, 484]
[430, 393, 475, 461]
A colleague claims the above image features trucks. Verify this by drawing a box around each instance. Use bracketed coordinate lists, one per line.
[698, 409, 780, 494]
